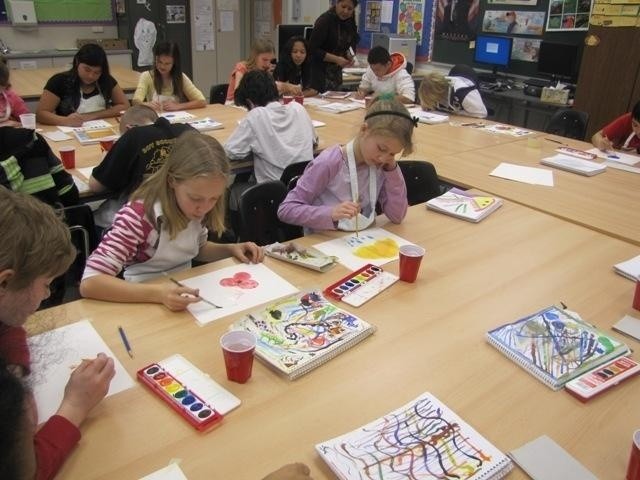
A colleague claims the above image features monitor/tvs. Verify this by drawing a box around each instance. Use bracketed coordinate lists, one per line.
[474, 36, 513, 73]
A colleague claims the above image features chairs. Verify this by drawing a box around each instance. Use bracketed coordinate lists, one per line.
[479, 90, 501, 118]
[395, 161, 442, 205]
[237, 181, 304, 247]
[449, 63, 479, 91]
[281, 162, 311, 191]
[52, 205, 99, 305]
[545, 108, 591, 141]
[209, 83, 229, 105]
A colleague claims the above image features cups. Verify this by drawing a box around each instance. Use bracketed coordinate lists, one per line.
[632, 274, 640, 312]
[296, 94, 304, 106]
[398, 245, 425, 283]
[626, 430, 640, 479]
[101, 138, 114, 161]
[283, 94, 294, 105]
[59, 146, 76, 169]
[220, 330, 257, 384]
[19, 113, 36, 129]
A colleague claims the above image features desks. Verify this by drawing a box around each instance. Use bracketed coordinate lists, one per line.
[225, 93, 549, 162]
[8, 64, 143, 113]
[341, 67, 425, 95]
[427, 134, 640, 245]
[447, 72, 573, 131]
[0, 188, 640, 480]
[0, 105, 345, 197]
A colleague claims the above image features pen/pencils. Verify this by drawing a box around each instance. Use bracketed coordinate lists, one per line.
[71, 106, 87, 124]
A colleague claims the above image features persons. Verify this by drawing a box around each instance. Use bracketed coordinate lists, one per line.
[223, 68, 319, 212]
[309, 0, 361, 93]
[418, 72, 488, 119]
[0, 362, 36, 480]
[0, 183, 116, 480]
[88, 104, 201, 194]
[506, 12, 519, 33]
[443, 1, 470, 37]
[0, 51, 34, 123]
[591, 99, 640, 151]
[272, 35, 322, 97]
[79, 129, 264, 312]
[131, 39, 207, 111]
[355, 45, 415, 104]
[36, 43, 131, 127]
[399, 3, 422, 42]
[276, 93, 419, 237]
[225, 42, 302, 106]
[0, 115, 80, 211]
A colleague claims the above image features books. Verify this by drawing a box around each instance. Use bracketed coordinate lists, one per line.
[611, 314, 640, 342]
[425, 187, 503, 223]
[227, 285, 377, 382]
[485, 303, 632, 391]
[540, 153, 608, 177]
[172, 117, 224, 132]
[318, 101, 360, 114]
[73, 125, 121, 145]
[314, 390, 514, 480]
[409, 110, 449, 125]
[600, 160, 640, 174]
[506, 434, 598, 480]
[41, 130, 74, 142]
[612, 255, 640, 282]
[320, 90, 352, 99]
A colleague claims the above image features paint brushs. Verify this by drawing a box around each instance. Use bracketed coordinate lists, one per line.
[117, 325, 133, 358]
[161, 271, 222, 308]
[604, 134, 616, 156]
[158, 94, 159, 114]
[544, 137, 568, 146]
[355, 192, 359, 238]
